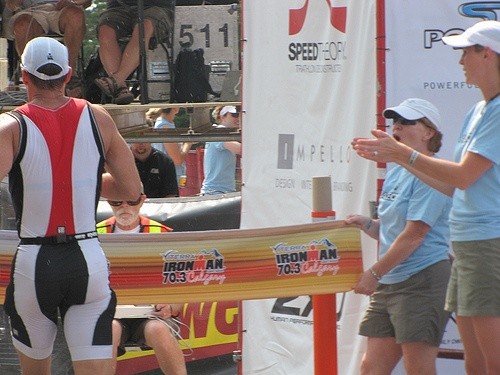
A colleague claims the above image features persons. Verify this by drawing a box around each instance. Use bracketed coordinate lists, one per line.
[95, 0, 175, 105]
[0, 0, 94, 98]
[198, 105, 242, 196]
[0, 36, 139, 375]
[93, 181, 191, 375]
[128, 134, 179, 199]
[340, 97, 456, 375]
[351, 21, 500, 375]
[145, 108, 192, 190]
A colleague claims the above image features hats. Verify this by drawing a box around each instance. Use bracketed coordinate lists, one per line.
[220, 106, 238, 116]
[21, 37, 69, 80]
[382, 98, 443, 132]
[441, 20, 500, 54]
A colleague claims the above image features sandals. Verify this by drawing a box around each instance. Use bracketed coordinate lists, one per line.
[94, 76, 118, 97]
[115, 86, 134, 105]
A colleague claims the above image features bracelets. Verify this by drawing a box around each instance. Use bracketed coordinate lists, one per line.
[409, 149, 419, 167]
[364, 218, 371, 232]
[370, 268, 382, 280]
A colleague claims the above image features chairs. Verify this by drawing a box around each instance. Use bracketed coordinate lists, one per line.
[98, 0, 177, 103]
[8, 31, 87, 103]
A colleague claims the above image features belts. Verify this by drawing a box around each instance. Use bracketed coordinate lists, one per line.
[20, 231, 98, 245]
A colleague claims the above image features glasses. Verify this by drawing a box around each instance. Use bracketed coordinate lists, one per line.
[393, 118, 416, 125]
[108, 196, 140, 206]
[230, 113, 238, 117]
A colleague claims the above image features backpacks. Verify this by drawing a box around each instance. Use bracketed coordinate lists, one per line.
[173, 48, 221, 103]
